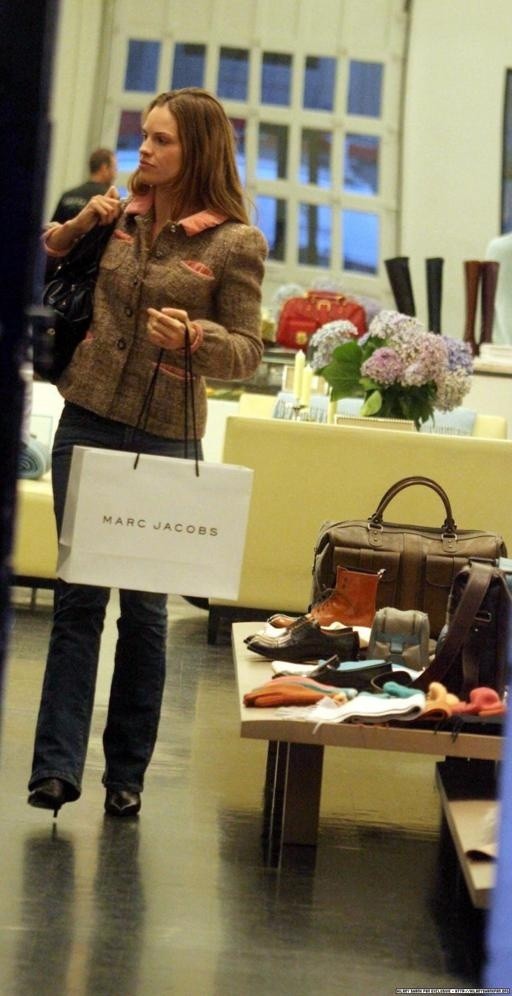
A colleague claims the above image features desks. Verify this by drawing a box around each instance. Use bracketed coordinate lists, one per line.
[228, 622, 504, 872]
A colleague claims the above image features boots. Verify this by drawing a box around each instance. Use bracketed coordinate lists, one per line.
[481, 258, 501, 343]
[383, 253, 417, 319]
[424, 255, 446, 335]
[462, 258, 483, 356]
[267, 563, 386, 641]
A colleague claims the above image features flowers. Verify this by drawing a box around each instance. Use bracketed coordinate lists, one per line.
[309, 308, 475, 432]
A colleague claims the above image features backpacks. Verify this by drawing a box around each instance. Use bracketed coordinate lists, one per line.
[369, 556, 512, 696]
[367, 606, 431, 671]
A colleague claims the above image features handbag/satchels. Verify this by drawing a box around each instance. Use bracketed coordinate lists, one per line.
[25, 218, 119, 383]
[275, 290, 369, 353]
[308, 475, 507, 641]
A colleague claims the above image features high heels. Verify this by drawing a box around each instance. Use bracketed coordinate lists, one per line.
[105, 787, 142, 817]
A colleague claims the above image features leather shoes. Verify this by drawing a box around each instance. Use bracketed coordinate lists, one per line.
[245, 614, 361, 663]
[26, 776, 68, 817]
[271, 655, 394, 694]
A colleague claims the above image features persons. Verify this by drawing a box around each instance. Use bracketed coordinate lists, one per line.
[25, 83, 269, 822]
[31, 149, 119, 384]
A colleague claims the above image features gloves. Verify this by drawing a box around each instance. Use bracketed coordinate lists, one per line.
[54, 320, 255, 607]
[243, 674, 358, 708]
